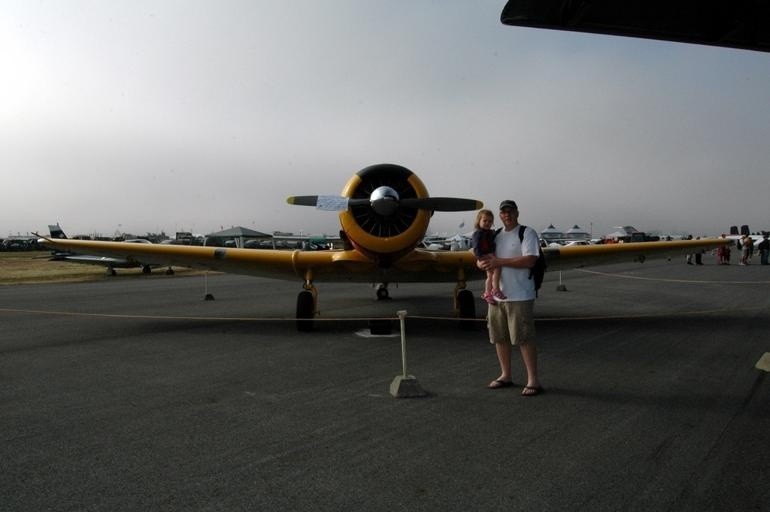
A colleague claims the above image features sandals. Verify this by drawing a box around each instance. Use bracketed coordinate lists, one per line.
[481, 289, 508, 303]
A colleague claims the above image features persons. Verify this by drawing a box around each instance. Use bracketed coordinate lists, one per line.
[684, 233, 770, 268]
[475, 199, 544, 395]
[472, 208, 508, 306]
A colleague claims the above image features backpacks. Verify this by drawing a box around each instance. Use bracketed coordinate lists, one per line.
[495, 225, 546, 290]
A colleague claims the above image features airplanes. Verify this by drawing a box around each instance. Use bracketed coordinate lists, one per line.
[718, 235, 770, 257]
[48, 223, 174, 277]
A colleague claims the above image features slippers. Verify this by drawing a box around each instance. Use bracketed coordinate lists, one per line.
[487, 377, 512, 389]
[521, 384, 541, 396]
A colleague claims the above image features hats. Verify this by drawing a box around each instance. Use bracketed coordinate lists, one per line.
[499, 200, 517, 210]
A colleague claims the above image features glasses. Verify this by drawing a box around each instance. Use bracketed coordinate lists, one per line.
[500, 210, 514, 214]
